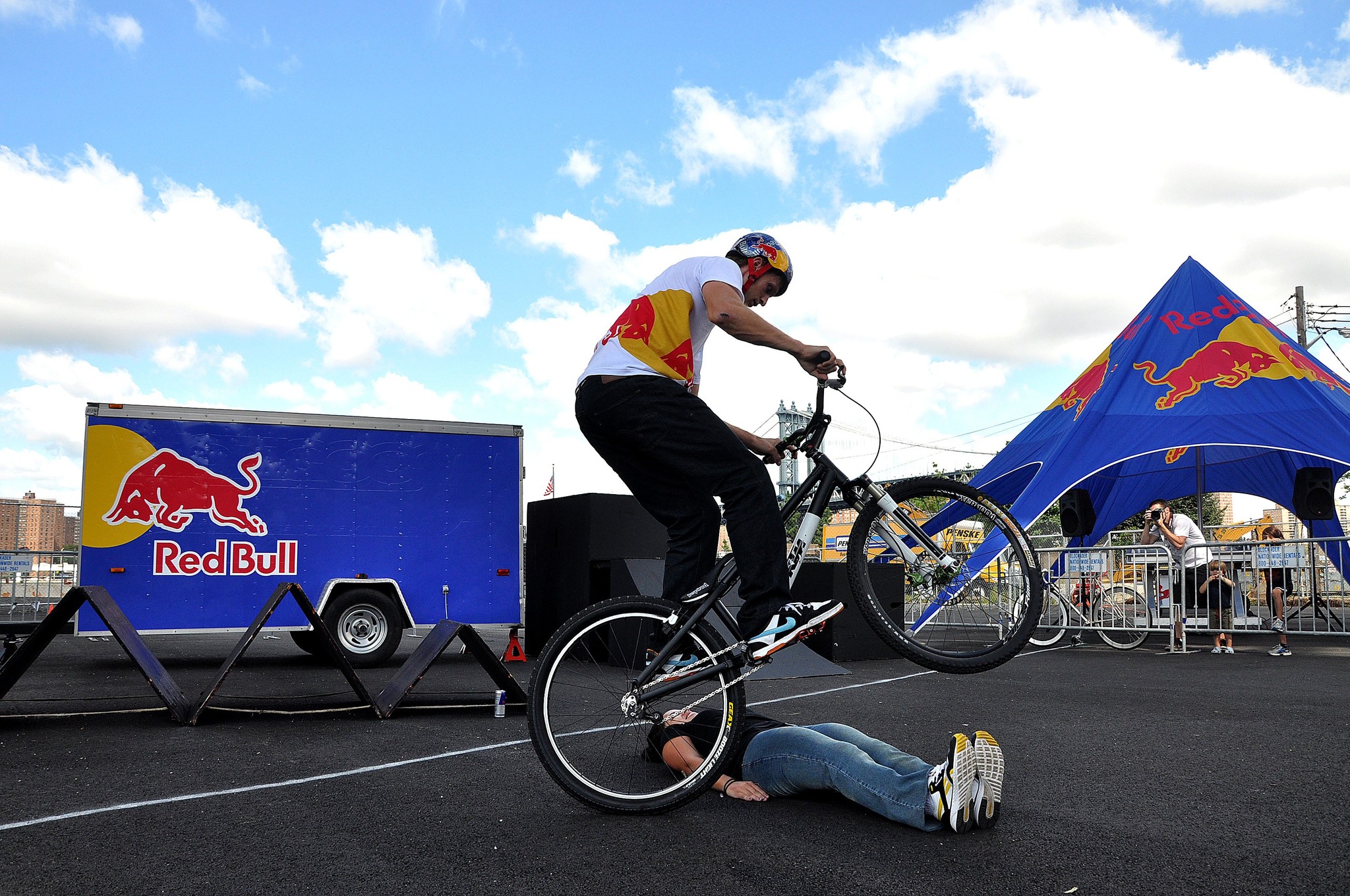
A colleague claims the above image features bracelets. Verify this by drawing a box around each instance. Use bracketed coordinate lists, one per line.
[720, 778, 737, 797]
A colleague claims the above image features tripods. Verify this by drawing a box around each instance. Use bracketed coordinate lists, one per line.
[1282, 520, 1349, 638]
[1045, 537, 1096, 633]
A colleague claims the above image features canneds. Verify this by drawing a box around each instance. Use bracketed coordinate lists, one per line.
[494, 690, 506, 718]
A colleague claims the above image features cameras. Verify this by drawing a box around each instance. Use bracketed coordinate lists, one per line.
[1150, 509, 1163, 520]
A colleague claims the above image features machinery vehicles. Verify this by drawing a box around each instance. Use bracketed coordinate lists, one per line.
[1112, 513, 1277, 598]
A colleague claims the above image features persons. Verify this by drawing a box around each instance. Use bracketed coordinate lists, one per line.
[648, 710, 1004, 830]
[575, 234, 848, 677]
[1141, 500, 1291, 656]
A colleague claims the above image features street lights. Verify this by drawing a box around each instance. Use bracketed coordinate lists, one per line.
[927, 462, 929, 475]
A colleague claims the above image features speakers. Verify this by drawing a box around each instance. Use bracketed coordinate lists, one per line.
[1059, 488, 1096, 538]
[1293, 467, 1334, 520]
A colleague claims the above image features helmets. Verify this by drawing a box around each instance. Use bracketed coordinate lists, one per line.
[725, 233, 793, 297]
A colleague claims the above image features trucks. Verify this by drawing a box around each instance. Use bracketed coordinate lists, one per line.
[821, 519, 1014, 600]
[0, 401, 522, 668]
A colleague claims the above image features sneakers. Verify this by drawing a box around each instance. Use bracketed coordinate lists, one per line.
[970, 731, 1005, 828]
[646, 634, 713, 682]
[928, 733, 977, 833]
[1165, 637, 1182, 651]
[747, 599, 844, 659]
[1268, 644, 1292, 656]
[1271, 618, 1285, 632]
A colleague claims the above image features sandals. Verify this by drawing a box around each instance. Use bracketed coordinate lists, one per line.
[1225, 647, 1234, 654]
[1211, 647, 1222, 654]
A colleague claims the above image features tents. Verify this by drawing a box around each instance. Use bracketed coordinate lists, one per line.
[869, 256, 1350, 640]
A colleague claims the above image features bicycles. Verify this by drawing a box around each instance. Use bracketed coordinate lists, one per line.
[522, 349, 1047, 820]
[1011, 568, 1153, 651]
